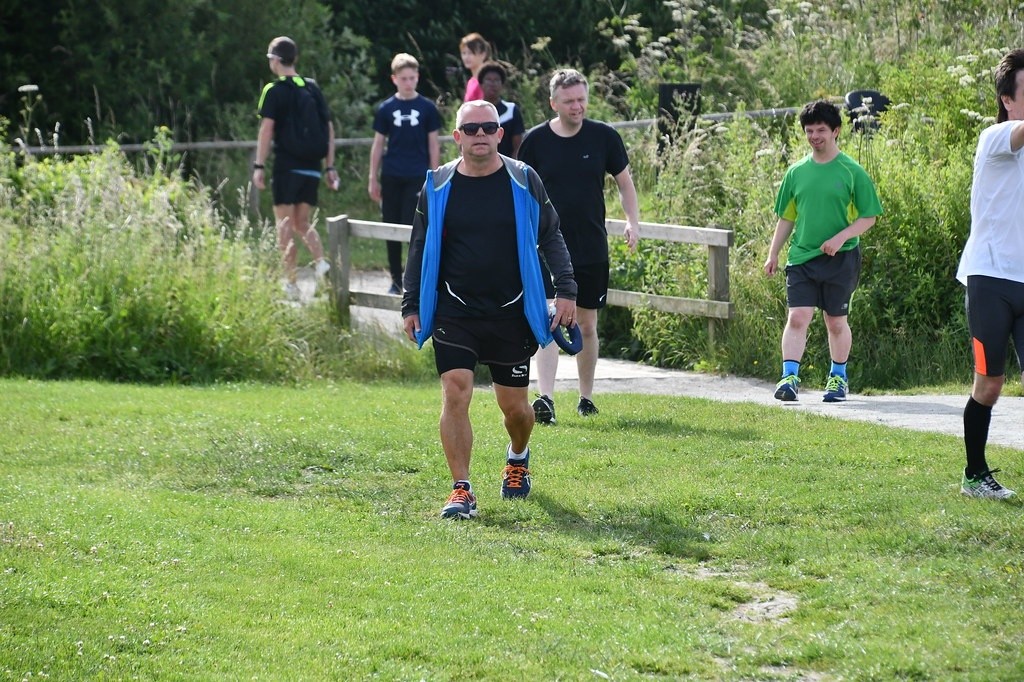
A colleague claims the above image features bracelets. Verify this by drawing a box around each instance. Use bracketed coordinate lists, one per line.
[326, 167, 335, 171]
[253, 164, 265, 169]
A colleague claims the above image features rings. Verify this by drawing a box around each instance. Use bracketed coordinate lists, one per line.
[568, 317, 573, 319]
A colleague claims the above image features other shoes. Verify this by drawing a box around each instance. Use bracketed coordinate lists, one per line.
[313, 261, 331, 297]
[389, 282, 403, 297]
[282, 285, 301, 300]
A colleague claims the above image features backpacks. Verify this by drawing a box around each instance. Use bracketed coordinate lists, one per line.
[274, 79, 329, 160]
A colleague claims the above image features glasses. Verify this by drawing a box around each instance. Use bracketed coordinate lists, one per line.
[458, 122, 499, 136]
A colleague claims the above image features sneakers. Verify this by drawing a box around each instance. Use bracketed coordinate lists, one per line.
[774, 372, 802, 401]
[823, 372, 849, 401]
[578, 396, 596, 416]
[531, 394, 556, 424]
[440, 482, 477, 519]
[960, 466, 1019, 503]
[500, 441, 531, 498]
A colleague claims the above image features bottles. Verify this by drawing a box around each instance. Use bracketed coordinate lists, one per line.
[548, 302, 583, 356]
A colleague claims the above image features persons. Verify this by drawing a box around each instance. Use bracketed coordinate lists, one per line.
[764, 102, 883, 402]
[368, 53, 445, 294]
[401, 100, 578, 520]
[517, 69, 640, 425]
[252, 36, 341, 301]
[955, 49, 1024, 506]
[477, 64, 526, 159]
[460, 33, 493, 103]
